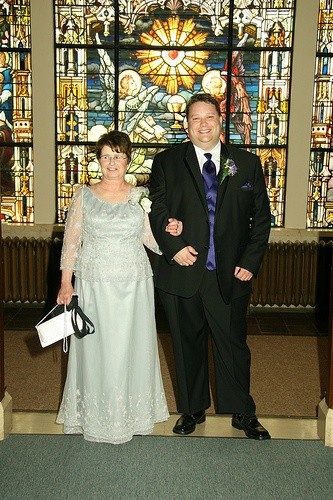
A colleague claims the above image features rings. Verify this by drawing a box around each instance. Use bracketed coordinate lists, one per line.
[175, 221, 179, 233]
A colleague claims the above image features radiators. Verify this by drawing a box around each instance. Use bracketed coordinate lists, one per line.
[0, 236, 333, 308]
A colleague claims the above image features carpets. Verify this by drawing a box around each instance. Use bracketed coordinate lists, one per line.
[0, 433, 333, 500]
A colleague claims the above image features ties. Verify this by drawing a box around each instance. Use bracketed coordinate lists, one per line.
[201, 153, 218, 272]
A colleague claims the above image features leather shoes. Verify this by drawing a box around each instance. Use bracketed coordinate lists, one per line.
[231, 413, 271, 440]
[172, 408, 206, 435]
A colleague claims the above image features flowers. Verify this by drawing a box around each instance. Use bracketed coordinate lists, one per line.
[138, 192, 153, 214]
[219, 155, 240, 185]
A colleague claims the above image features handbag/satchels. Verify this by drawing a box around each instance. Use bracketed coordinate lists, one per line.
[35, 293, 86, 354]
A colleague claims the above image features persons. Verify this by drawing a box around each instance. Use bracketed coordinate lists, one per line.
[56, 130, 183, 444]
[148, 93, 272, 440]
